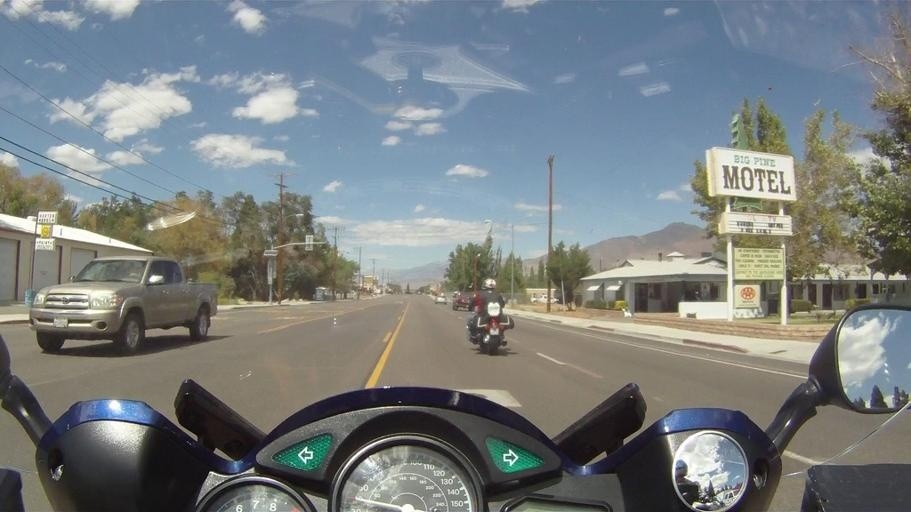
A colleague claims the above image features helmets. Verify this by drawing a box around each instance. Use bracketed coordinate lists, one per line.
[481, 277, 498, 291]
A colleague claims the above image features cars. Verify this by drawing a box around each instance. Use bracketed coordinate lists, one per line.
[452, 292, 476, 311]
[435, 296, 447, 304]
[723, 489, 740, 504]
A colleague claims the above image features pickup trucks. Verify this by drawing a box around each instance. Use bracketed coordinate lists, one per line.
[29, 256, 218, 355]
[530, 295, 560, 304]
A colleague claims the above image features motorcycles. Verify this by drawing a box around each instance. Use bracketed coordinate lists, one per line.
[465, 294, 514, 354]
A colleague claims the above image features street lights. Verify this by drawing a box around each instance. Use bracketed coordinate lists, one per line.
[483, 219, 514, 308]
[286, 213, 304, 220]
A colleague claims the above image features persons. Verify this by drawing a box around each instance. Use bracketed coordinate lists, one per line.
[467, 278, 507, 348]
